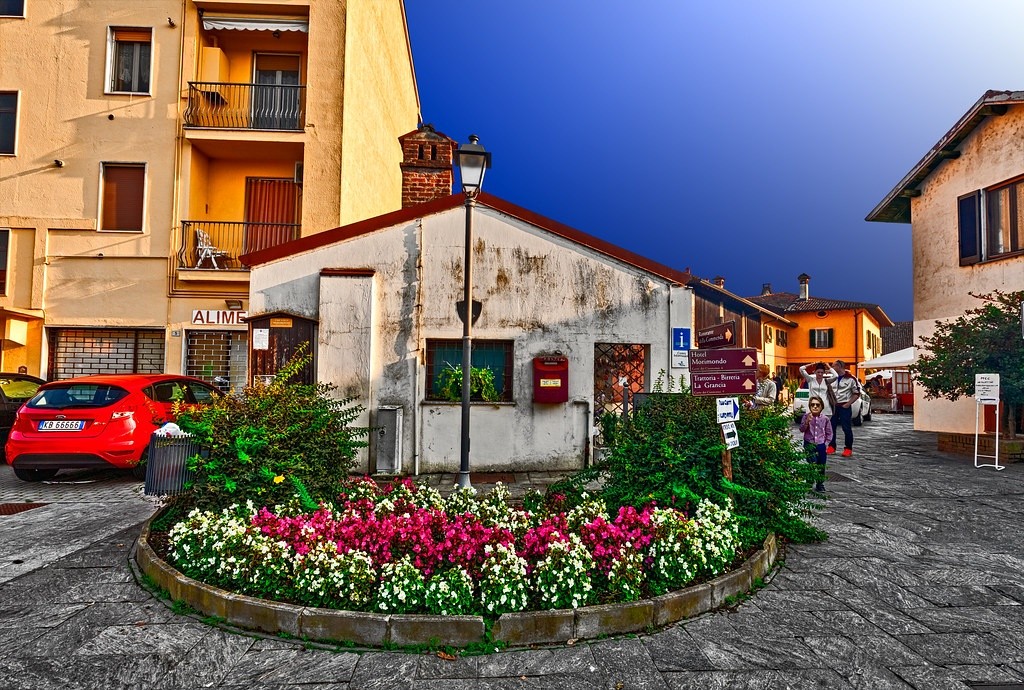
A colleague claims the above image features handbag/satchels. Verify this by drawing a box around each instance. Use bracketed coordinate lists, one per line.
[825, 378, 837, 406]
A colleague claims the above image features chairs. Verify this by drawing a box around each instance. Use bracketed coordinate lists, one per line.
[196, 228, 229, 270]
[157, 384, 198, 403]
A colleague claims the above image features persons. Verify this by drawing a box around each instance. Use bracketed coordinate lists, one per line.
[871, 375, 892, 388]
[798, 397, 833, 493]
[798, 361, 839, 420]
[826, 360, 862, 456]
[771, 372, 781, 400]
[743, 364, 776, 410]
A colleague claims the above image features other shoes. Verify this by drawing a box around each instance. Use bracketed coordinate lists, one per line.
[805, 480, 813, 488]
[816, 483, 826, 493]
[841, 448, 852, 457]
[826, 446, 836, 455]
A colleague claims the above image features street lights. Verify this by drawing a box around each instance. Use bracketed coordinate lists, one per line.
[450, 133, 492, 499]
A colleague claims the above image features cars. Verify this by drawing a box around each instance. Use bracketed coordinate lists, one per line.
[0, 372, 83, 467]
[793, 368, 872, 426]
[5, 373, 234, 480]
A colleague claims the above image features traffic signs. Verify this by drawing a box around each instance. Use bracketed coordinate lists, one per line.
[715, 397, 741, 424]
[688, 347, 758, 373]
[721, 421, 740, 451]
[690, 373, 758, 396]
[697, 320, 736, 349]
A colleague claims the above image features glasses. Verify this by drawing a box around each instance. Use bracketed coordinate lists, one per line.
[810, 403, 821, 408]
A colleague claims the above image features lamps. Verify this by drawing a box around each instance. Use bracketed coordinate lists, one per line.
[273, 32, 280, 39]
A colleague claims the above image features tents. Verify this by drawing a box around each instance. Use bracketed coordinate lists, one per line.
[857, 347, 914, 368]
[865, 370, 892, 384]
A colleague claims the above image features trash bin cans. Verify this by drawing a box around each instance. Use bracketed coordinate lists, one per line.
[144, 431, 196, 498]
